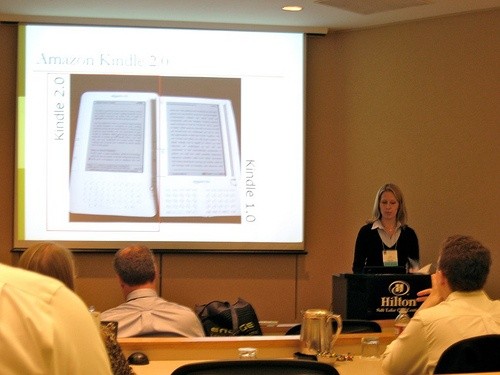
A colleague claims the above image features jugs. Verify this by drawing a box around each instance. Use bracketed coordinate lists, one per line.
[298, 308, 343, 357]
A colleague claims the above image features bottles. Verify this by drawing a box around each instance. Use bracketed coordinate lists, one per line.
[393, 309, 410, 338]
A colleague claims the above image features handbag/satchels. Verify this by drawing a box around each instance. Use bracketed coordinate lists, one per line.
[196, 297, 262, 337]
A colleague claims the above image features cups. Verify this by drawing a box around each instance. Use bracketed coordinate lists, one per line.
[238, 348, 256, 359]
[361, 337, 378, 359]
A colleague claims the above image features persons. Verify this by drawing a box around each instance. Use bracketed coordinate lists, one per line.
[99, 244, 204, 338]
[0, 264, 112, 374]
[14, 241, 77, 291]
[381, 235, 500, 375]
[352, 184, 420, 274]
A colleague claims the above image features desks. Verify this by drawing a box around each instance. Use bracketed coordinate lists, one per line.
[117, 334, 397, 374]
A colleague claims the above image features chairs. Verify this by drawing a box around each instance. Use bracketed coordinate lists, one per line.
[172, 359, 341, 375]
[433, 333, 500, 375]
[284, 320, 381, 335]
